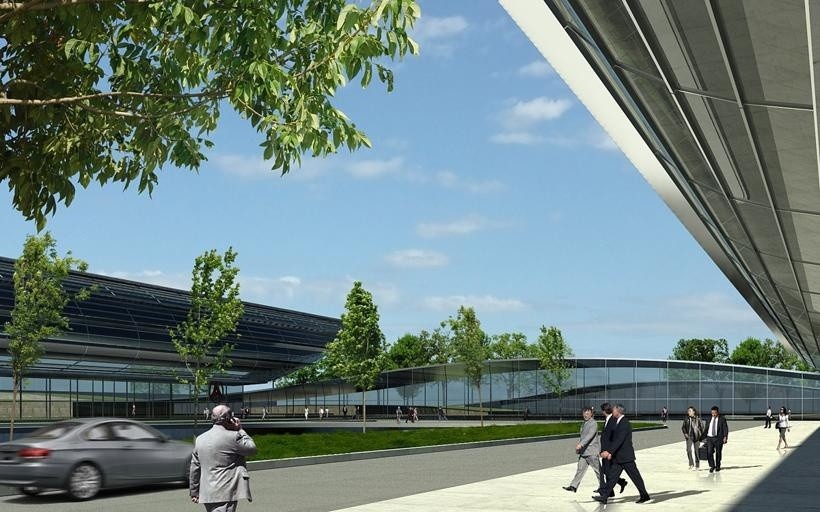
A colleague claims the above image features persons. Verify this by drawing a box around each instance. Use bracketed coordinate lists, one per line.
[764, 405, 792, 450]
[661, 406, 728, 473]
[131, 402, 135, 416]
[396, 405, 448, 423]
[305, 405, 360, 420]
[524, 405, 531, 420]
[204, 405, 250, 420]
[189, 405, 256, 512]
[562, 403, 650, 504]
[262, 406, 268, 419]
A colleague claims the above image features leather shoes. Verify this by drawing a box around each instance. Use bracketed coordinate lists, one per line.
[689, 464, 722, 473]
[563, 478, 651, 504]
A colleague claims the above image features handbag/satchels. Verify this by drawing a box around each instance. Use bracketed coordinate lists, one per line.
[775, 414, 780, 429]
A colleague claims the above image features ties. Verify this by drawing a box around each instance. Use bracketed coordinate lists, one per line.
[614, 420, 617, 430]
[712, 418, 715, 436]
[603, 419, 606, 428]
